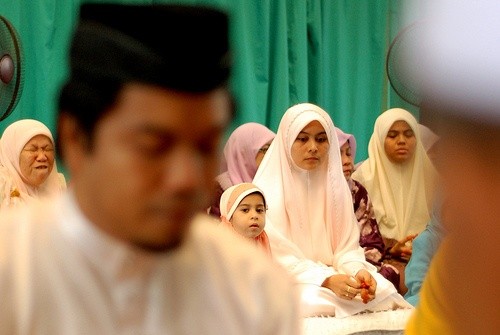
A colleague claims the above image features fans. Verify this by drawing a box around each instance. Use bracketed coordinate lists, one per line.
[386, 20, 426, 108]
[0, 16, 26, 122]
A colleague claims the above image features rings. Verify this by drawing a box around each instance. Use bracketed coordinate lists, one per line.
[345, 287, 350, 297]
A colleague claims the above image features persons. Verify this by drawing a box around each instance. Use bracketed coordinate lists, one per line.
[218, 182, 272, 256]
[0, 119, 66, 210]
[250, 102, 414, 319]
[332, 127, 400, 290]
[211, 123, 277, 221]
[351, 107, 474, 307]
[0, 0, 301, 335]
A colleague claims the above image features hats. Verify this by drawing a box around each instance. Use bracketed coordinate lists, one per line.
[220, 182, 268, 222]
[68, 2, 231, 96]
